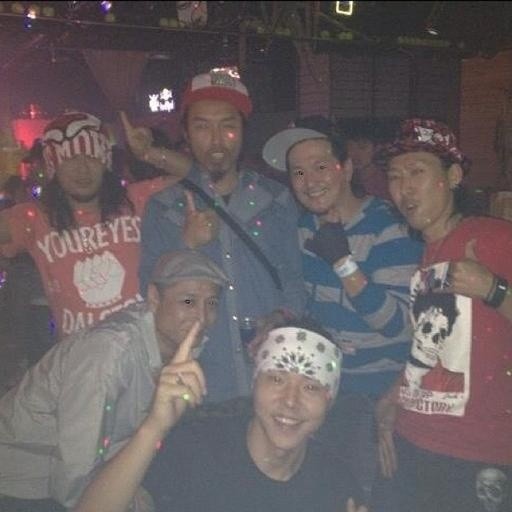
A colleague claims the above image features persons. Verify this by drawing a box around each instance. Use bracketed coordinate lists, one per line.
[2, 248, 233, 512]
[71, 310, 373, 511]
[368, 115, 511, 512]
[1, 108, 195, 346]
[259, 114, 425, 511]
[346, 117, 391, 200]
[135, 71, 310, 412]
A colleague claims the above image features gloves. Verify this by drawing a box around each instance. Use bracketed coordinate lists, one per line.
[305, 222, 351, 267]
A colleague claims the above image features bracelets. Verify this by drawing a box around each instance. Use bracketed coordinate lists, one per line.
[159, 146, 168, 172]
[483, 276, 506, 308]
[334, 261, 358, 279]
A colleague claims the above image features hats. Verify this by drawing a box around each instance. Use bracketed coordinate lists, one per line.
[182, 69, 252, 114]
[384, 117, 473, 167]
[150, 249, 231, 289]
[40, 110, 115, 171]
[337, 113, 382, 144]
[260, 114, 344, 171]
